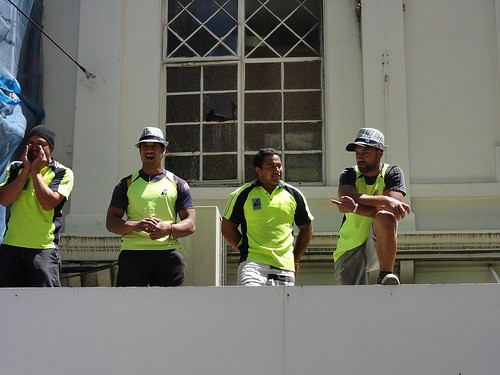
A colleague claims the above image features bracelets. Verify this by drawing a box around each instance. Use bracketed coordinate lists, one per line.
[353, 203, 359, 213]
[169, 224, 172, 244]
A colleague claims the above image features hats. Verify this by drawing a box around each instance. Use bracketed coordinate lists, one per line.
[346, 127, 385, 152]
[28, 125, 56, 150]
[135, 127, 169, 148]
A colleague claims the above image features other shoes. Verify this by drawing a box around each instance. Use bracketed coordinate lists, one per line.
[378, 270, 400, 285]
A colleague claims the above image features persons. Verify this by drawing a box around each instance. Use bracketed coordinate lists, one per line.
[222, 148, 315, 286]
[330, 127, 411, 285]
[105, 127, 198, 287]
[0, 124, 74, 288]
